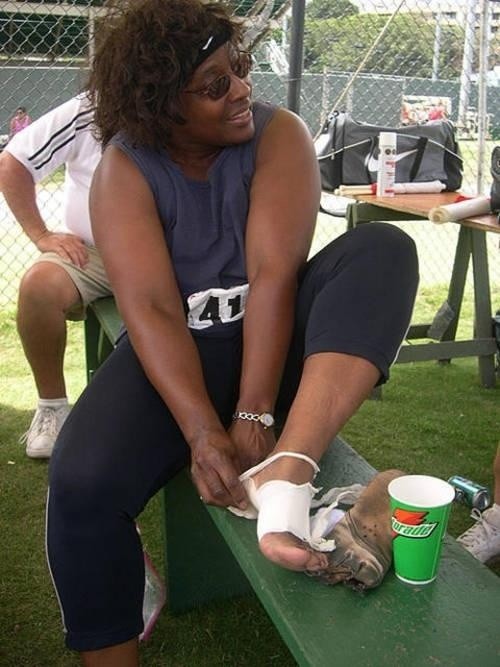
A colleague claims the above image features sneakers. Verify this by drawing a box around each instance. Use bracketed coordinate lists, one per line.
[19, 403, 71, 460]
[454, 504, 500, 564]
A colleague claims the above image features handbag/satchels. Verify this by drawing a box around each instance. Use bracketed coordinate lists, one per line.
[312, 109, 466, 191]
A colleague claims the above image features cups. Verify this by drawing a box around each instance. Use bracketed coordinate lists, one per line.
[387, 474, 455, 586]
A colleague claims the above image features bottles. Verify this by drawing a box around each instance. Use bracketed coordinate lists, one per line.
[376, 131, 397, 197]
[447, 474, 492, 511]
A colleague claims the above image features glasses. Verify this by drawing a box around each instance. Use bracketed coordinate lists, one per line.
[180, 48, 254, 103]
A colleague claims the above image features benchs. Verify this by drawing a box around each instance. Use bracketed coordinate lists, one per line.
[80, 296, 500, 667]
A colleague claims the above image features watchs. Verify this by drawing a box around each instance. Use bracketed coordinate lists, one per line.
[231, 409, 277, 430]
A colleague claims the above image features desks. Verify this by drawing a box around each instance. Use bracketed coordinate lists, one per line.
[321, 187, 500, 402]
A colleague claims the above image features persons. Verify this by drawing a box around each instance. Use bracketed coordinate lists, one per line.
[10, 107, 32, 139]
[0, 90, 114, 458]
[455, 440, 500, 564]
[45, 1, 420, 667]
